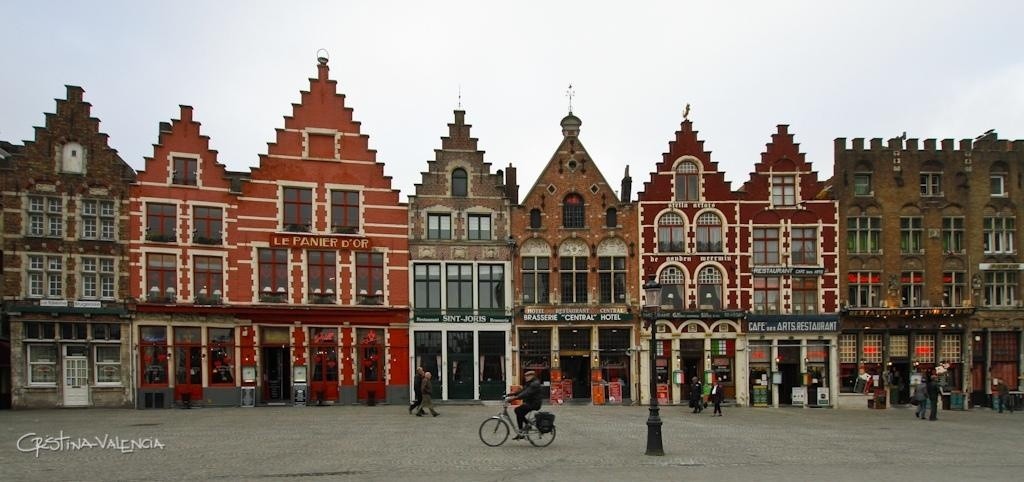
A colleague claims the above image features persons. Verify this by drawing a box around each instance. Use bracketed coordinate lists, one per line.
[708, 379, 724, 417]
[913, 377, 927, 419]
[504, 370, 543, 440]
[408, 366, 428, 416]
[996, 377, 1014, 413]
[927, 373, 943, 421]
[416, 372, 441, 417]
[689, 376, 703, 413]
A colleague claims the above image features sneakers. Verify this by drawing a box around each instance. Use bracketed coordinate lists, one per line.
[513, 435, 525, 440]
[916, 412, 926, 419]
[692, 408, 722, 417]
[408, 406, 440, 417]
[519, 425, 532, 431]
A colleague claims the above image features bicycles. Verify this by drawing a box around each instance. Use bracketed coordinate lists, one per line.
[478, 394, 555, 449]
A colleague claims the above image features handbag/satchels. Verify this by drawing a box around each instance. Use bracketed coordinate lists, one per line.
[536, 412, 554, 433]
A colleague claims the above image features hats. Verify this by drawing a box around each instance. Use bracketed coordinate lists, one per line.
[524, 371, 535, 376]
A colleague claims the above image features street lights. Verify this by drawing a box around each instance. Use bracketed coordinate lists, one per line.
[638, 273, 667, 456]
[122, 296, 139, 407]
